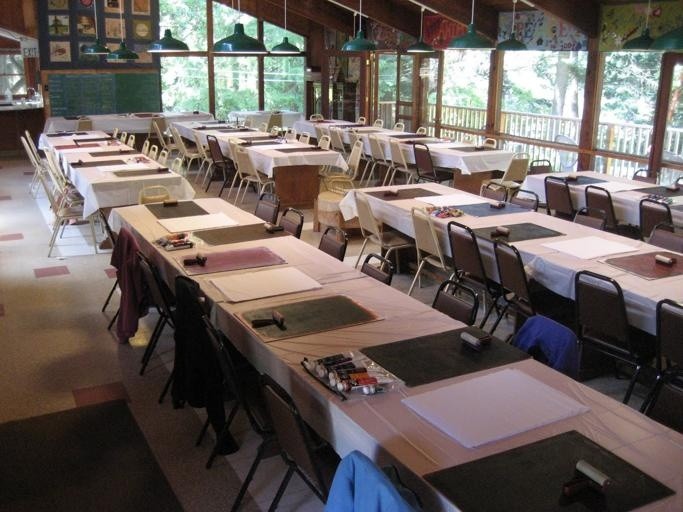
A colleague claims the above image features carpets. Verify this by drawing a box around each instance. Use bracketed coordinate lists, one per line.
[0, 399, 183, 512]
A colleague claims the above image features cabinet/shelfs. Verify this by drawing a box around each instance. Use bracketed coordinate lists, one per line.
[0, 92, 45, 160]
[312, 80, 357, 121]
